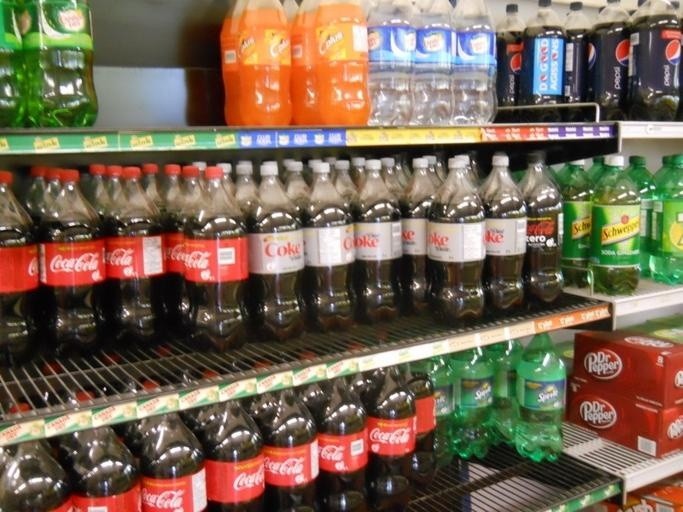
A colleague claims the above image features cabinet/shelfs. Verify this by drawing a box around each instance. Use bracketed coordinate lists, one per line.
[0, 102, 683, 512]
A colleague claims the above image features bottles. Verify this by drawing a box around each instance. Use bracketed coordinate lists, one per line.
[3, 329, 565, 510]
[0, 154, 682, 363]
[0, 0, 683, 128]
